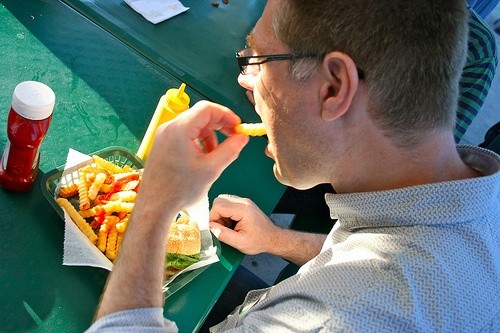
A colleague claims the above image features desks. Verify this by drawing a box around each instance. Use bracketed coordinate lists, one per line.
[0, 1, 287, 333]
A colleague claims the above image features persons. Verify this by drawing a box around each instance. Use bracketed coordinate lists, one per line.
[81, 0, 500, 333]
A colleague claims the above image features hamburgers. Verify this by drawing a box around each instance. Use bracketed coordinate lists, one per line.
[161, 210, 201, 275]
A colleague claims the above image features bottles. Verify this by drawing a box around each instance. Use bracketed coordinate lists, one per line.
[137, 83, 190, 162]
[0, 81, 55, 191]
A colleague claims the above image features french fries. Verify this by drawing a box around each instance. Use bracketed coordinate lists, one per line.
[56, 155, 143, 262]
[235, 122, 267, 136]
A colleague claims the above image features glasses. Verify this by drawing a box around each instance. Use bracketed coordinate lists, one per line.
[235, 47, 366, 81]
